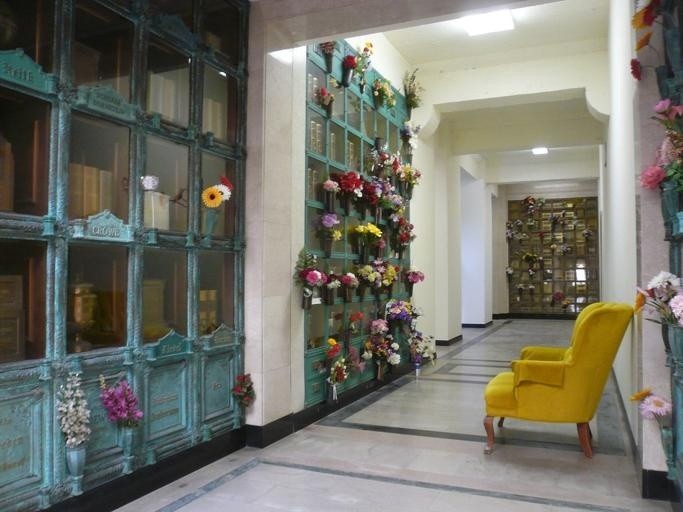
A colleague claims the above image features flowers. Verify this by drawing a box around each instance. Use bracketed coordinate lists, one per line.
[232, 376, 255, 427]
[56, 370, 91, 448]
[507, 196, 603, 308]
[98, 374, 145, 427]
[629, 0, 683, 429]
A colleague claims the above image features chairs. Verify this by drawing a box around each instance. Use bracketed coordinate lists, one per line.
[483, 300, 635, 457]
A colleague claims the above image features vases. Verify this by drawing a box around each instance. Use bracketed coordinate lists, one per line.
[65, 447, 88, 497]
[119, 424, 137, 476]
[658, 430, 675, 466]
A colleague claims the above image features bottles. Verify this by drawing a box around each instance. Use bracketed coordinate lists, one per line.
[67, 283, 98, 326]
[197, 289, 219, 332]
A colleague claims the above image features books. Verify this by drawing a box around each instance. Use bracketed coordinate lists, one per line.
[70, 161, 116, 220]
[197, 290, 222, 334]
[307, 71, 356, 204]
[70, 47, 226, 139]
[140, 281, 166, 327]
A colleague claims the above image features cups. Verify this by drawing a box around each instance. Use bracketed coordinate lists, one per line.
[144, 189, 170, 231]
